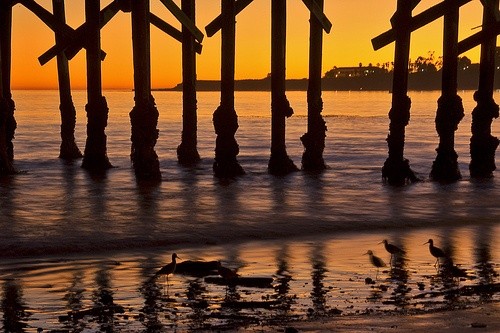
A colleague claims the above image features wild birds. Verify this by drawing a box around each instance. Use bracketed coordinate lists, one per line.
[361, 248, 388, 280]
[377, 238, 409, 268]
[216, 258, 241, 293]
[442, 260, 471, 288]
[422, 237, 448, 273]
[152, 252, 182, 288]
